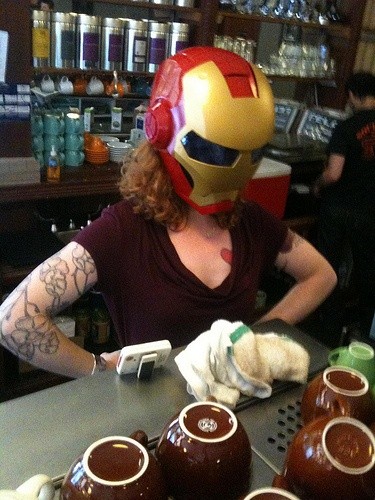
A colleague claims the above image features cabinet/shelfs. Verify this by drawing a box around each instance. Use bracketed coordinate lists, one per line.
[0, 160, 127, 403]
[0, 0, 366, 219]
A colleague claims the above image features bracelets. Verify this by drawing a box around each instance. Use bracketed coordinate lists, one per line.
[89, 354, 108, 376]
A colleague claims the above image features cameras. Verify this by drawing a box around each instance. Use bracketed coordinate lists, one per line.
[116, 340, 172, 379]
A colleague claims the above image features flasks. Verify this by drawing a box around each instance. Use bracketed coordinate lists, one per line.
[32, 0, 196, 74]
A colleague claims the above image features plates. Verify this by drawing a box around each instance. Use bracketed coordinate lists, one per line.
[85, 135, 132, 164]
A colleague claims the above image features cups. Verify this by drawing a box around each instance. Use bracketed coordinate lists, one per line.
[59, 430, 166, 500]
[298, 366, 375, 427]
[155, 395, 252, 500]
[31, 113, 85, 168]
[328, 342, 375, 395]
[284, 415, 375, 500]
[239, 475, 300, 500]
[40, 74, 152, 97]
[0, 473, 55, 500]
[214, 0, 337, 79]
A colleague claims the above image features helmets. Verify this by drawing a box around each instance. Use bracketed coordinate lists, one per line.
[143, 46, 275, 217]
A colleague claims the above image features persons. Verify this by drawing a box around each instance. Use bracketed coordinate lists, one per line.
[0, 47, 339, 379]
[312, 71, 375, 346]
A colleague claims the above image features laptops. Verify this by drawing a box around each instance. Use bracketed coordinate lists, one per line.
[228, 318, 331, 413]
